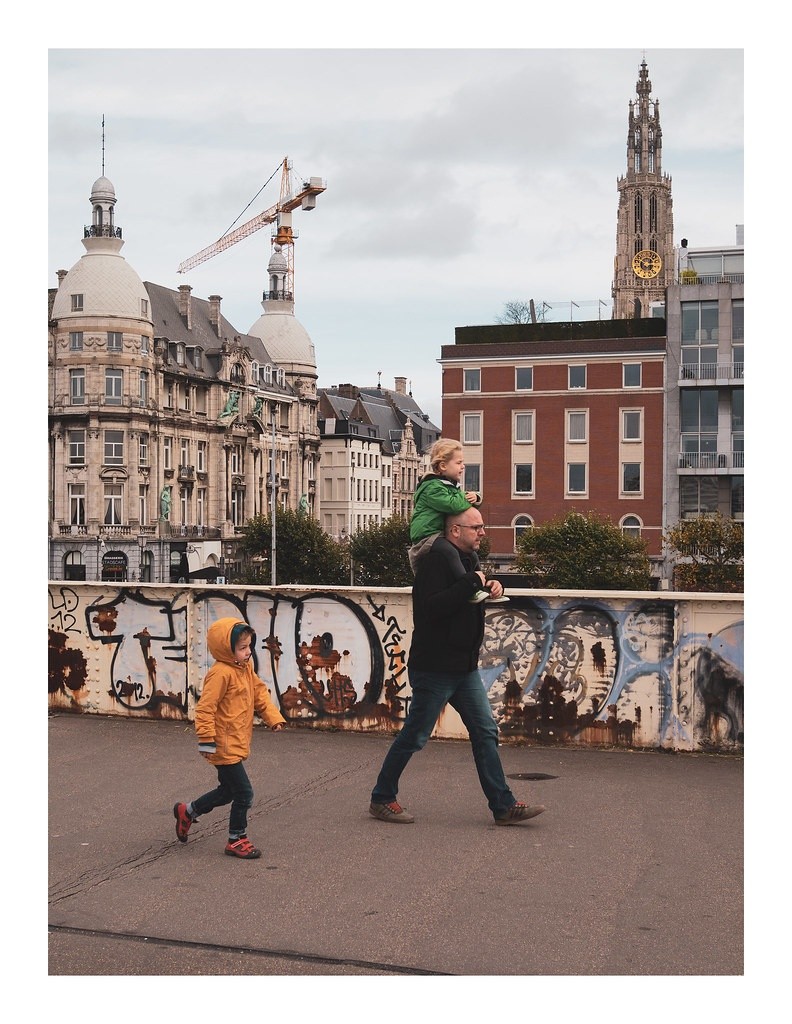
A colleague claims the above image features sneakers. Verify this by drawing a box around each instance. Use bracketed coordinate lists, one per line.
[495, 801, 546, 826]
[369, 801, 414, 823]
[173, 802, 199, 843]
[225, 832, 262, 859]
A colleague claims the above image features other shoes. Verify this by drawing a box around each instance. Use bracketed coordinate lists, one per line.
[467, 589, 489, 604]
[484, 595, 510, 604]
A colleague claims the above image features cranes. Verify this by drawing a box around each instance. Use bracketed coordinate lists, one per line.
[175, 154, 327, 300]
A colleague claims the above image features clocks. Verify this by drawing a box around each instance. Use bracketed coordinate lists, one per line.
[632, 249, 662, 278]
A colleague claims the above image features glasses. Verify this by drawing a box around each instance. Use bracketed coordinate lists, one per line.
[454, 524, 486, 532]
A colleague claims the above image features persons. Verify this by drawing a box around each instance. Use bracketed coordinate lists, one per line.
[409, 438, 510, 603]
[368, 507, 547, 825]
[174, 616, 287, 858]
[298, 494, 310, 512]
[253, 396, 263, 416]
[220, 391, 240, 418]
[159, 485, 172, 520]
